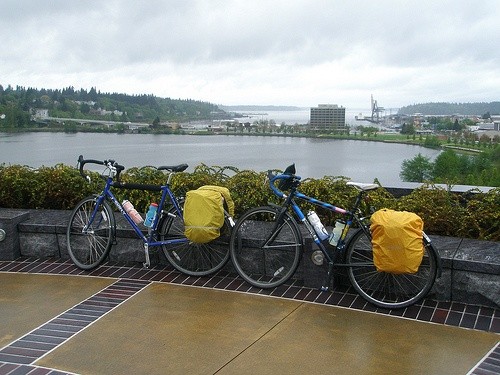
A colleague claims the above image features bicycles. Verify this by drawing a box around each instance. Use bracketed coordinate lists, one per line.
[65, 155, 244, 277]
[230, 169, 443, 310]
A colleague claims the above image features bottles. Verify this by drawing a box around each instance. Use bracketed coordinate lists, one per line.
[329, 218, 346, 246]
[307, 210, 329, 240]
[121, 199, 144, 226]
[143, 202, 158, 227]
